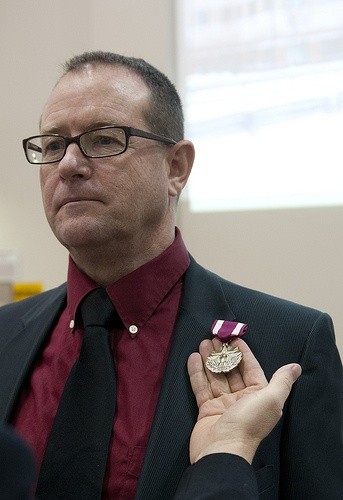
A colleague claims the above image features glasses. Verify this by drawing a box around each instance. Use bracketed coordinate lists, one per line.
[20, 124, 177, 165]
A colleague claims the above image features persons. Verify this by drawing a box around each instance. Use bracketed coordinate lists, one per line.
[1, 51, 343, 500]
[0, 338, 302, 500]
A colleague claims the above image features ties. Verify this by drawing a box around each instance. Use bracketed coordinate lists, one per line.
[34, 291, 118, 500]
